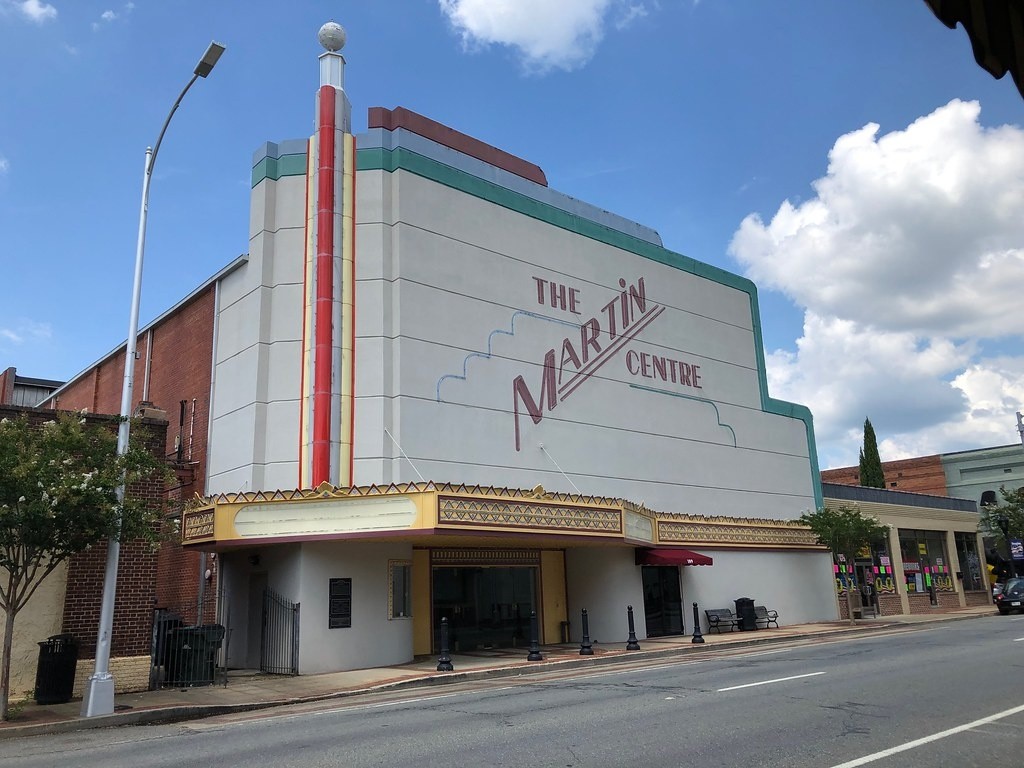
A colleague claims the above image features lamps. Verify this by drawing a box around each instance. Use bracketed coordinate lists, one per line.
[1004, 468, 1012, 474]
[891, 482, 897, 486]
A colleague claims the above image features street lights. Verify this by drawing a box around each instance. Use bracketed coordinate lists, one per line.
[997, 514, 1017, 577]
[87, 38, 233, 714]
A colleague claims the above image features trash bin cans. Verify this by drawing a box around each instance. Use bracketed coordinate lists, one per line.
[33, 634, 79, 705]
[734, 597, 756, 631]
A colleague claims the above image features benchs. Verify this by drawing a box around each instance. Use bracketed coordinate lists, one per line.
[847, 592, 877, 620]
[754, 605, 779, 630]
[705, 608, 743, 634]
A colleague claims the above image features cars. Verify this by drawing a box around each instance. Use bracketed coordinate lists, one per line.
[995, 577, 1023, 615]
